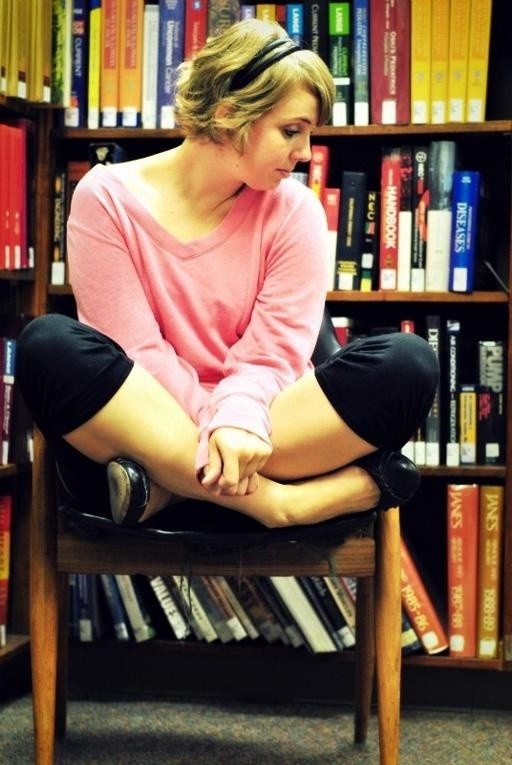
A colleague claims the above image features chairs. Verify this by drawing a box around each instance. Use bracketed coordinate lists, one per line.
[22, 289, 408, 765]
[22, 99, 408, 765]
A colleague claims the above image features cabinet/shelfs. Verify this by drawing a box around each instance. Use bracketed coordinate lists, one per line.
[0, 1, 56, 710]
[45, 287, 512, 476]
[64, 467, 511, 671]
[60, 3, 510, 131]
[42, 121, 510, 301]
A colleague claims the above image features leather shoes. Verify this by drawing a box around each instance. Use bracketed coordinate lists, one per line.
[265, 449, 421, 533]
[107, 457, 150, 528]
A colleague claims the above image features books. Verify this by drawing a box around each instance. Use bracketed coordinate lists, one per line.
[52, 141, 127, 284]
[68, 536, 448, 655]
[332, 316, 504, 466]
[0, 120, 35, 269]
[292, 142, 489, 294]
[0, 0, 503, 128]
[0, 313, 33, 466]
[449, 485, 502, 658]
[0, 497, 29, 648]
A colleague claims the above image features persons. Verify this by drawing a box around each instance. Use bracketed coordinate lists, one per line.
[15, 17, 439, 534]
